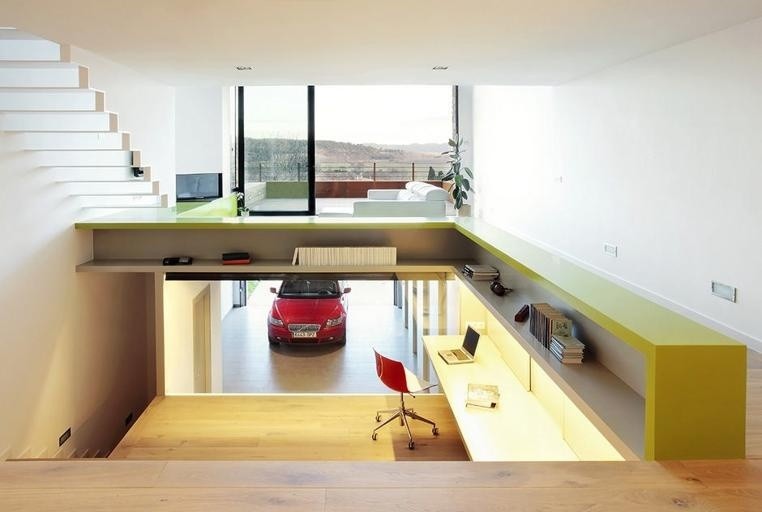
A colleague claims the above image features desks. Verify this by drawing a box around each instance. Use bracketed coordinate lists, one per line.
[419, 333, 585, 462]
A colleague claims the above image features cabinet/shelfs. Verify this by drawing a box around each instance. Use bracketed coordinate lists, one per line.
[457, 223, 751, 461]
[67, 213, 457, 277]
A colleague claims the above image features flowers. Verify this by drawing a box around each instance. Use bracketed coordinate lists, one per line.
[236, 191, 250, 212]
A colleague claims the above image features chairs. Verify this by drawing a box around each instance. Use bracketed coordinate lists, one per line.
[368, 342, 441, 452]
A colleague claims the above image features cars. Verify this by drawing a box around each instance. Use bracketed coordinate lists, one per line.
[267, 279, 353, 345]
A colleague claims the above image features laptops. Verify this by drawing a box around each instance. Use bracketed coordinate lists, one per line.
[438, 324, 481, 364]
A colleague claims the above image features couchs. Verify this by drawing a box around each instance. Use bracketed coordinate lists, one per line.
[350, 178, 453, 216]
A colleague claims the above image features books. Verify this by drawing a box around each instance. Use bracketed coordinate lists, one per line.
[529, 302, 585, 365]
[292, 247, 397, 265]
[464, 264, 499, 281]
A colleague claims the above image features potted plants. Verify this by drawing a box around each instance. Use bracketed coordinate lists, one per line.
[437, 133, 474, 217]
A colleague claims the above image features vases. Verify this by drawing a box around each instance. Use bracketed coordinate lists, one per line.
[241, 211, 250, 217]
